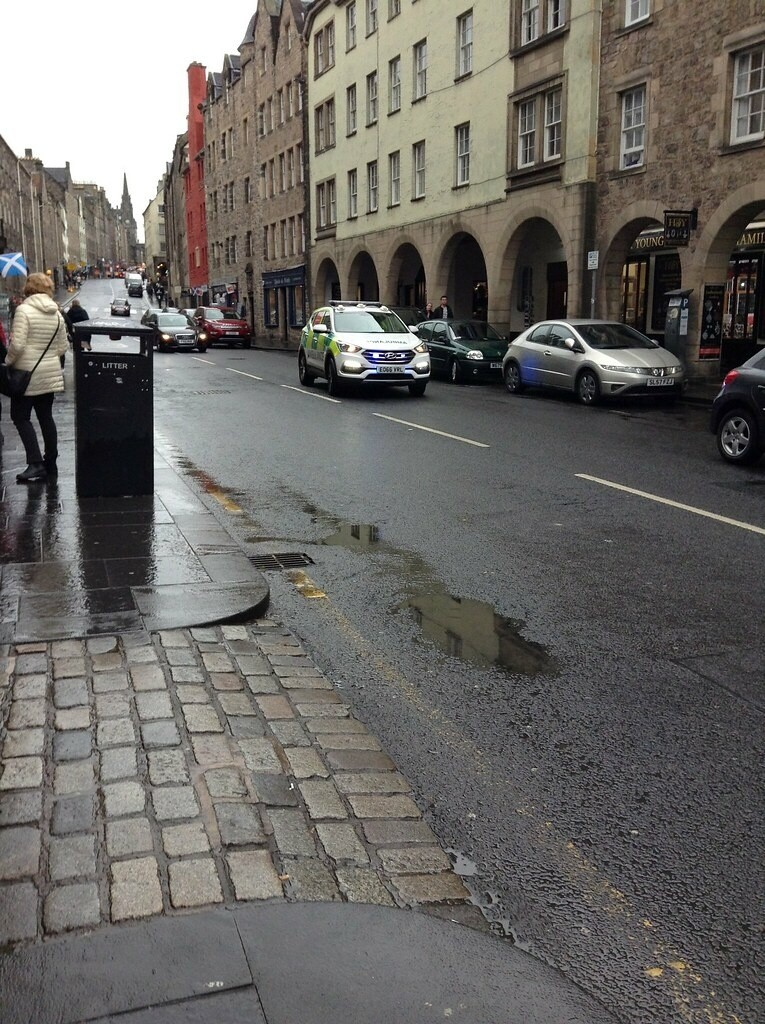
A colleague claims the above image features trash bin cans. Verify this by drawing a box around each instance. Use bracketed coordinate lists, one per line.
[73, 317, 154, 495]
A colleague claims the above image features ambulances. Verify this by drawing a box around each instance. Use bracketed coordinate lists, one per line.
[299, 300, 430, 397]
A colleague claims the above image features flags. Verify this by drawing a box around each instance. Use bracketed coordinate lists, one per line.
[0, 253, 26, 278]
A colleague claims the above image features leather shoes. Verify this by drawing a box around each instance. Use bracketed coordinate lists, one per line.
[15, 462, 58, 480]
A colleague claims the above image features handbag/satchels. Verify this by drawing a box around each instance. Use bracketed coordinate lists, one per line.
[0, 365, 31, 401]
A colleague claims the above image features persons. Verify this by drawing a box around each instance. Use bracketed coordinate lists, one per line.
[4, 272, 69, 481]
[66, 300, 91, 350]
[8, 295, 22, 319]
[146, 279, 167, 308]
[73, 275, 81, 289]
[434, 296, 453, 319]
[142, 272, 145, 285]
[65, 276, 68, 288]
[421, 302, 433, 320]
[55, 301, 68, 369]
[168, 297, 174, 307]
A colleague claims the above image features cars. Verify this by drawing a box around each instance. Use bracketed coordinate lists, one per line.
[110, 299, 132, 317]
[502, 319, 688, 407]
[414, 319, 509, 385]
[141, 313, 207, 353]
[192, 306, 251, 350]
[125, 271, 144, 298]
[711, 341, 765, 464]
[141, 307, 196, 325]
